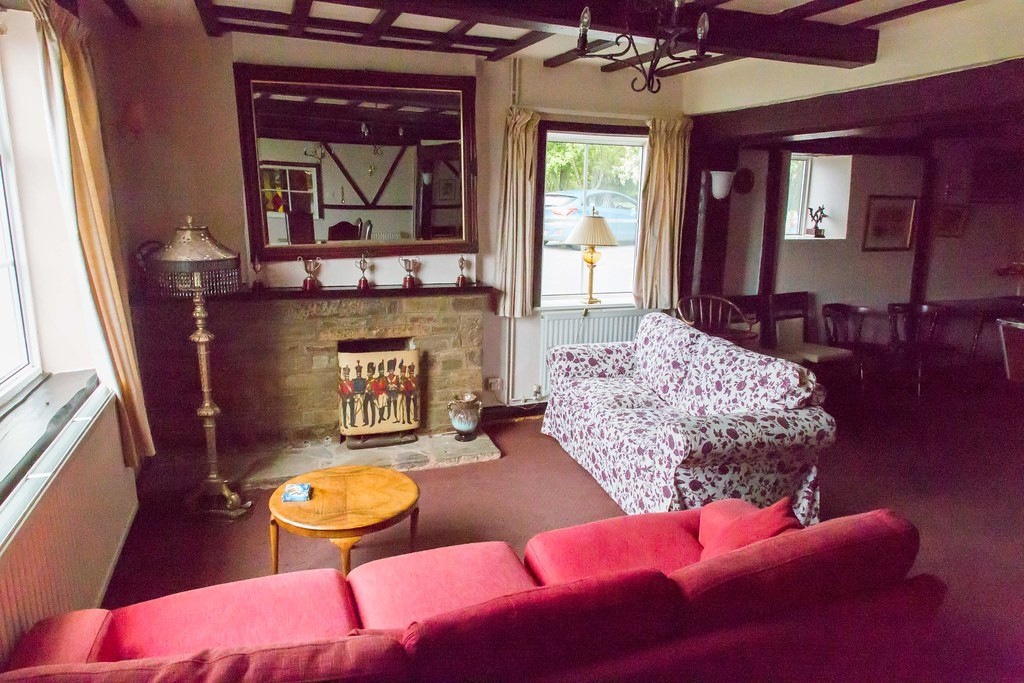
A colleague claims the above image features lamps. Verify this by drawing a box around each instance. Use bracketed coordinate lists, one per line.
[146, 215, 252, 519]
[564, 206, 618, 304]
[710, 168, 754, 200]
[572, 0, 712, 94]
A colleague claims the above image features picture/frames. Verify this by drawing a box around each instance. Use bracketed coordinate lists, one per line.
[439, 179, 457, 202]
[861, 194, 917, 252]
[935, 202, 972, 239]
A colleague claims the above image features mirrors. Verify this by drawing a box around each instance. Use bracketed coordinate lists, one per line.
[233, 62, 479, 262]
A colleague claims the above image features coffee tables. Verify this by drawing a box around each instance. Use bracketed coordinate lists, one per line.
[269, 466, 420, 576]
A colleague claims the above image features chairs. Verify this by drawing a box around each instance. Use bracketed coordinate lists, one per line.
[822, 303, 901, 402]
[768, 291, 853, 363]
[996, 318, 1024, 383]
[677, 295, 804, 364]
[327, 218, 373, 240]
[887, 303, 948, 398]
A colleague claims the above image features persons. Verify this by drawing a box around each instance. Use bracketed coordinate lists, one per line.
[338, 358, 421, 429]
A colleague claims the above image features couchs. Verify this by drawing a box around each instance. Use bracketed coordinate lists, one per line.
[540, 312, 837, 528]
[0, 499, 948, 683]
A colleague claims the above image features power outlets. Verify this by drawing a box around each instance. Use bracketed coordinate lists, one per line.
[485, 377, 500, 391]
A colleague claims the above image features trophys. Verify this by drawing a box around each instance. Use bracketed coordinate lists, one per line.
[355, 253, 372, 291]
[296, 253, 321, 291]
[247, 250, 269, 292]
[398, 255, 420, 288]
[456, 255, 468, 287]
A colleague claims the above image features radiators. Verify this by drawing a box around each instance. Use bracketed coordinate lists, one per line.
[537, 308, 663, 398]
[0, 382, 139, 665]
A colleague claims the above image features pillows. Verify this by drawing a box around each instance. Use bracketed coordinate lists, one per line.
[699, 495, 800, 563]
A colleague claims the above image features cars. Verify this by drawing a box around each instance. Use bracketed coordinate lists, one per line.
[543, 189, 638, 250]
